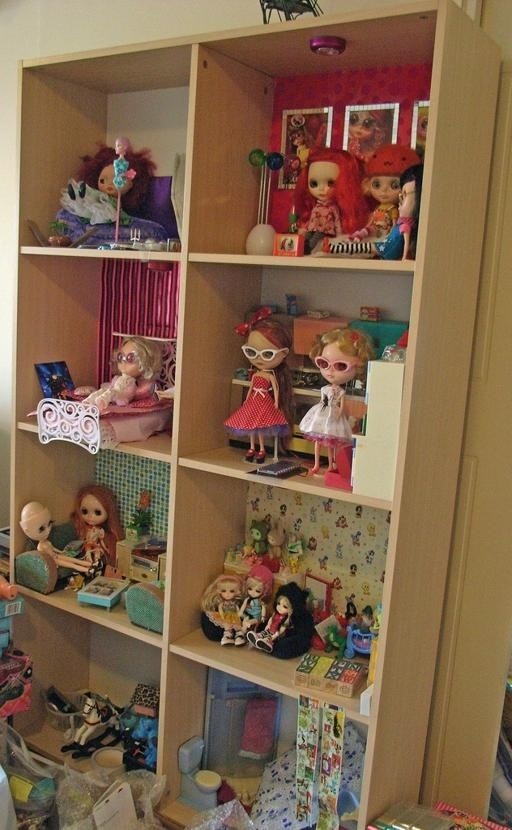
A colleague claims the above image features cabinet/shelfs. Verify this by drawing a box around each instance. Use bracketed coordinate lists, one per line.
[3, 2, 510, 830]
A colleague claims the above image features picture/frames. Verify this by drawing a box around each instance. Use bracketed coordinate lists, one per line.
[273, 97, 427, 195]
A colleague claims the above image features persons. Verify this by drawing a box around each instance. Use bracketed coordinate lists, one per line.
[81, 336, 162, 409]
[20, 485, 126, 582]
[201, 566, 308, 657]
[0, 107, 424, 777]
[286, 144, 424, 260]
[60, 137, 158, 243]
[225, 306, 371, 475]
